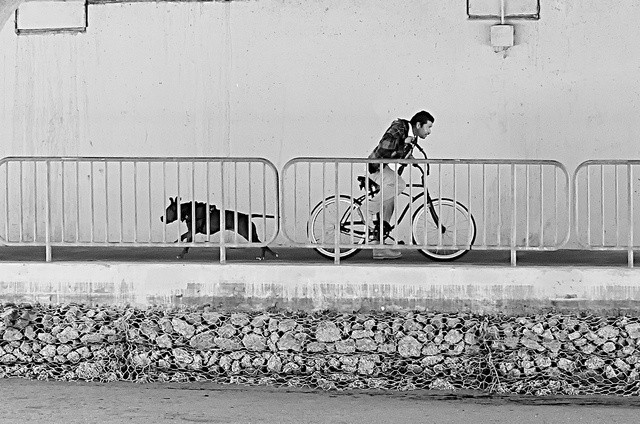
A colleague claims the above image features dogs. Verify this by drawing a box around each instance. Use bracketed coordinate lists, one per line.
[160, 197, 279, 260]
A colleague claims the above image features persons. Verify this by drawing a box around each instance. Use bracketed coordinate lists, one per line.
[357, 110, 434, 259]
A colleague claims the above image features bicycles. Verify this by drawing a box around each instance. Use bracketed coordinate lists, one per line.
[308, 164, 477, 262]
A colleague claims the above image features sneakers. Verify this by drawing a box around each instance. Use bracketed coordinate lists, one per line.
[356, 205, 377, 229]
[374, 248, 403, 260]
